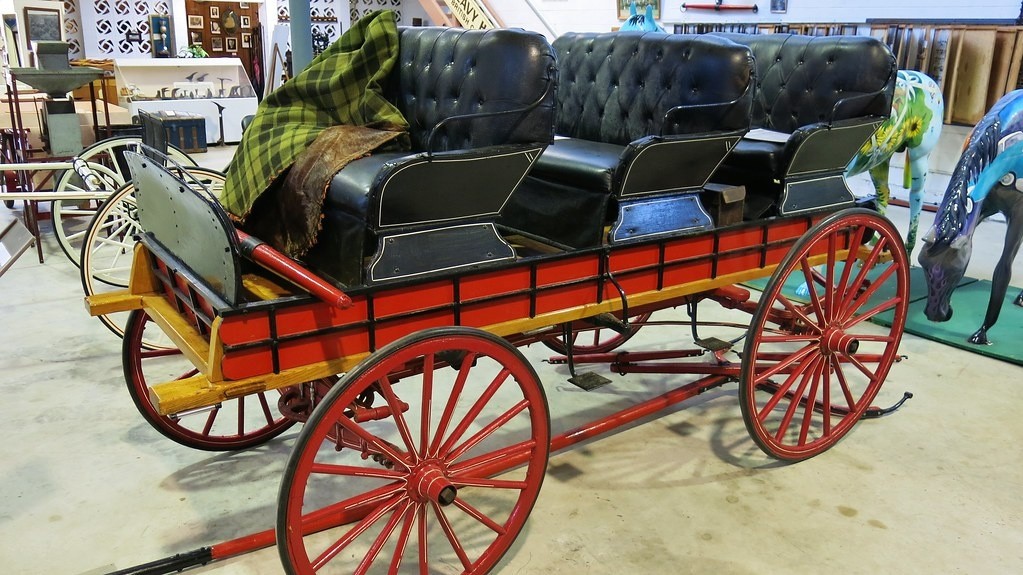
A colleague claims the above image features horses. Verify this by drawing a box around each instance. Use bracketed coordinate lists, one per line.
[842, 71, 944, 266]
[617, 1, 668, 35]
[917, 90, 1022, 346]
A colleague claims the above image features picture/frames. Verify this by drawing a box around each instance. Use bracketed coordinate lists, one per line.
[769, 0, 787, 13]
[187, 2, 253, 53]
[23, 6, 62, 50]
[617, 0, 661, 20]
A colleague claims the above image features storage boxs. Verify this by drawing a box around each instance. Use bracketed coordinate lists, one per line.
[73, 98, 130, 147]
[151, 111, 208, 153]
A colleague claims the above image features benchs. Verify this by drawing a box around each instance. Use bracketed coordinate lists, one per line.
[243, 26, 558, 285]
[699, 33, 899, 219]
[494, 32, 757, 251]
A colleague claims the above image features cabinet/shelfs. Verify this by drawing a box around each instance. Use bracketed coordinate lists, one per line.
[5, 71, 126, 266]
